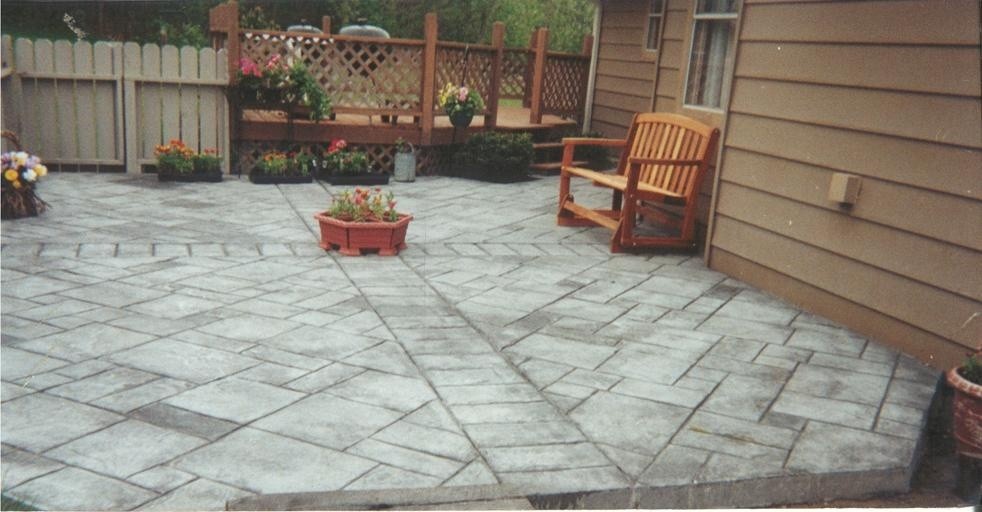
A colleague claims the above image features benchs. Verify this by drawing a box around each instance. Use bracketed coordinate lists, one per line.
[558, 110, 721, 254]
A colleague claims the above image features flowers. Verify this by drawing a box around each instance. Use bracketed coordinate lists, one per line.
[437, 81, 489, 115]
[151, 140, 223, 174]
[326, 185, 399, 222]
[958, 348, 981, 381]
[234, 53, 331, 126]
[0, 150, 49, 189]
[248, 139, 371, 175]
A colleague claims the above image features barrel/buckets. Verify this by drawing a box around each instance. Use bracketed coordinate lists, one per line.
[393, 140, 416, 181]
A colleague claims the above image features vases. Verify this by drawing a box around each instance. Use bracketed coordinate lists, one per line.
[248, 168, 389, 186]
[448, 111, 474, 128]
[158, 171, 222, 181]
[313, 211, 414, 257]
[944, 365, 982, 458]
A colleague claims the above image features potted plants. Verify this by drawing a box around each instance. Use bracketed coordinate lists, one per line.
[557, 129, 608, 167]
[464, 130, 534, 181]
[392, 135, 418, 182]
